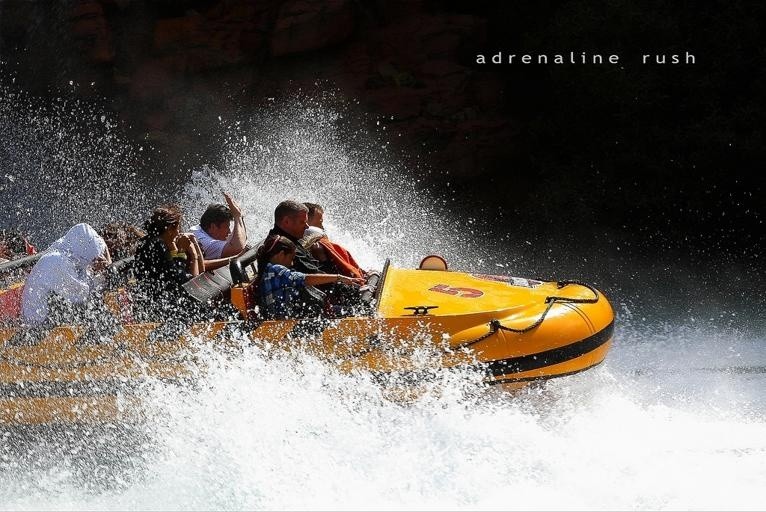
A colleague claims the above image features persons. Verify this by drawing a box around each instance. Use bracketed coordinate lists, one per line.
[0, 192, 368, 322]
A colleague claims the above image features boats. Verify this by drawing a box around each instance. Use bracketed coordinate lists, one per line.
[0, 231, 615, 424]
